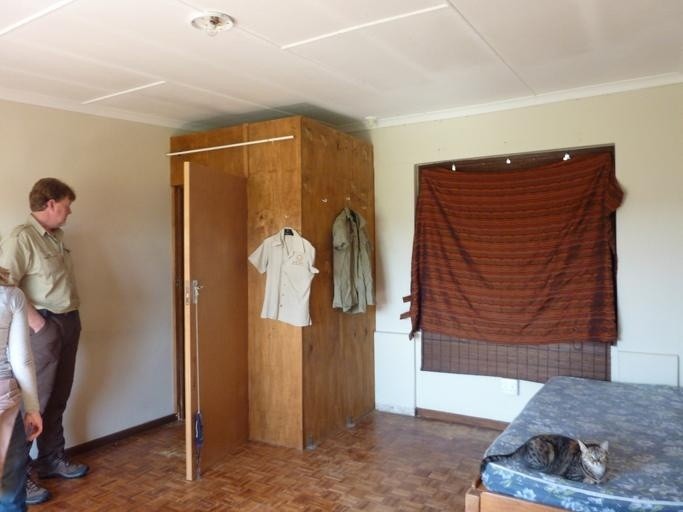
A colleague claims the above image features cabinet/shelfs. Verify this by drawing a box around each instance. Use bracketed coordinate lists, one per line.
[161, 116, 375, 480]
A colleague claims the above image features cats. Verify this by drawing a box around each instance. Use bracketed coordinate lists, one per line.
[471, 433, 610, 490]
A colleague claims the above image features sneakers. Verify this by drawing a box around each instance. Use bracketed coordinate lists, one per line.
[23, 476, 50, 504]
[35, 455, 89, 478]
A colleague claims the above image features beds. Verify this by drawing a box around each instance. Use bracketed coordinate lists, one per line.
[463, 373, 683, 512]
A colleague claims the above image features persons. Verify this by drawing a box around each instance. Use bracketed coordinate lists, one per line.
[0, 178, 89, 504]
[0, 263, 45, 512]
